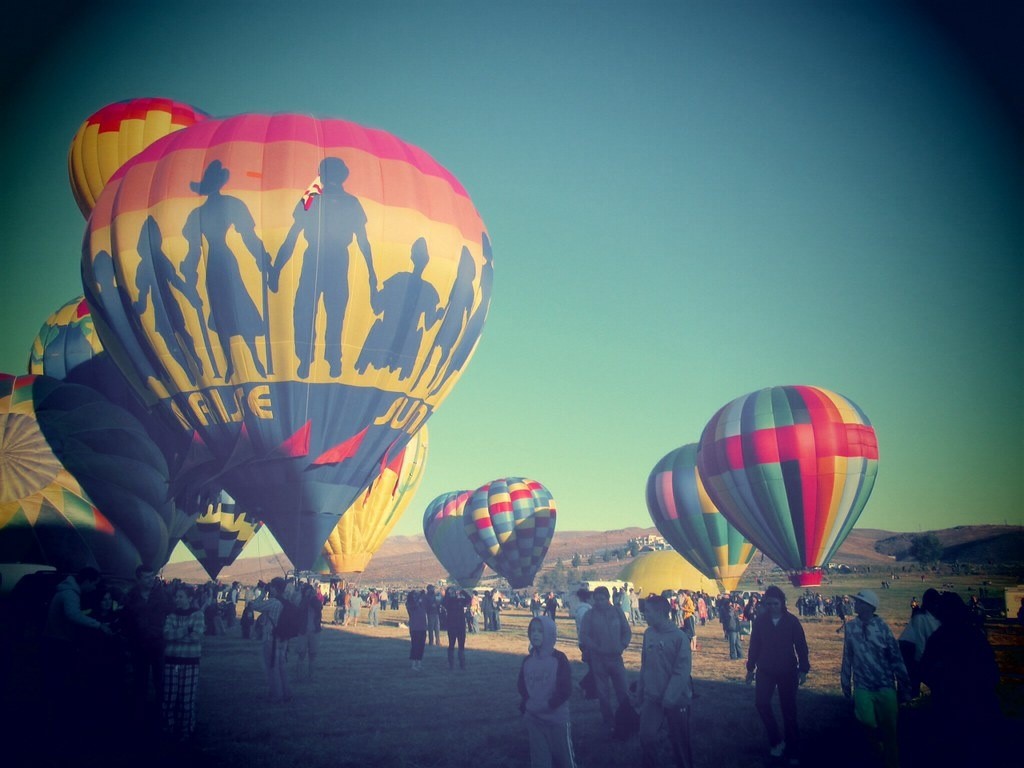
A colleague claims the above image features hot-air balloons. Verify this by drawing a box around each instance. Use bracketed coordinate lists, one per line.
[79, 110, 497, 595]
[0, 369, 175, 609]
[421, 488, 488, 589]
[460, 476, 558, 608]
[612, 549, 721, 599]
[317, 424, 429, 598]
[692, 384, 880, 589]
[27, 294, 224, 588]
[179, 490, 265, 588]
[642, 440, 759, 597]
[64, 97, 214, 225]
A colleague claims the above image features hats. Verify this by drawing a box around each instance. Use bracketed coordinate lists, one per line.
[847, 591, 878, 607]
[427, 585, 435, 593]
[233, 581, 239, 586]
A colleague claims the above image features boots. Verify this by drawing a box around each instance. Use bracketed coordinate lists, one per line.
[411, 659, 417, 671]
[418, 659, 423, 669]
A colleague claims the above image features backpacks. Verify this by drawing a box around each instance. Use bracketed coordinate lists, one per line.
[269, 594, 300, 641]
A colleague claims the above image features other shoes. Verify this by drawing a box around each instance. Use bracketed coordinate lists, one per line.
[790, 757, 798, 765]
[771, 739, 785, 756]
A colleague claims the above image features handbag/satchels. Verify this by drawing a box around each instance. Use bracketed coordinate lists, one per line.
[615, 701, 641, 739]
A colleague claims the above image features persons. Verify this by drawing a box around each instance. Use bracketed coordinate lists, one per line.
[161, 585, 205, 744]
[911, 597, 919, 611]
[881, 581, 890, 589]
[636, 596, 693, 768]
[839, 591, 906, 768]
[331, 584, 560, 673]
[251, 577, 292, 705]
[725, 600, 746, 660]
[161, 578, 331, 643]
[794, 588, 852, 635]
[613, 575, 765, 637]
[578, 587, 632, 724]
[897, 588, 951, 709]
[293, 584, 322, 684]
[746, 585, 811, 764]
[518, 616, 577, 768]
[45, 562, 156, 668]
[943, 582, 954, 589]
